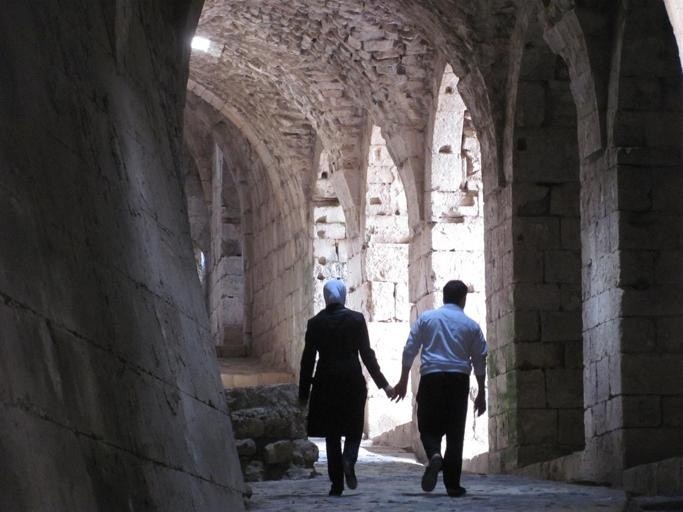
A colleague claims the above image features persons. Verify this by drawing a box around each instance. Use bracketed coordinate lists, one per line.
[390, 280, 489, 498]
[293, 279, 397, 495]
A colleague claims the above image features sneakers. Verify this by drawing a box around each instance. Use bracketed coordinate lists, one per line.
[421, 453, 442, 491]
[447, 486, 466, 497]
[329, 461, 357, 496]
